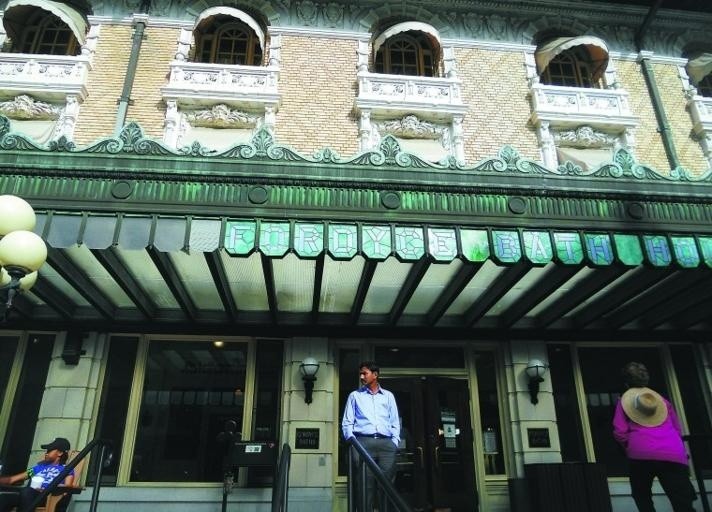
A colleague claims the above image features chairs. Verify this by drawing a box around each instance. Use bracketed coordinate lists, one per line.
[19, 450, 85, 511]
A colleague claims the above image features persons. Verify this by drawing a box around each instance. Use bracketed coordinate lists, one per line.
[1, 437, 76, 512]
[342, 361, 401, 512]
[611, 360, 698, 512]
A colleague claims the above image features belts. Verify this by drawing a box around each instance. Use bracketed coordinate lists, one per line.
[356, 432, 393, 439]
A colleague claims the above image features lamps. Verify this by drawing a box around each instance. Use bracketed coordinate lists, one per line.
[526, 359, 545, 407]
[297, 355, 321, 405]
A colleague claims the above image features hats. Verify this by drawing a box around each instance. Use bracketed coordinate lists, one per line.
[41, 437, 70, 450]
[621, 386, 668, 427]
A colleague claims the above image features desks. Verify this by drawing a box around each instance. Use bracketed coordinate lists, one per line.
[482, 452, 500, 475]
[0, 492, 19, 512]
